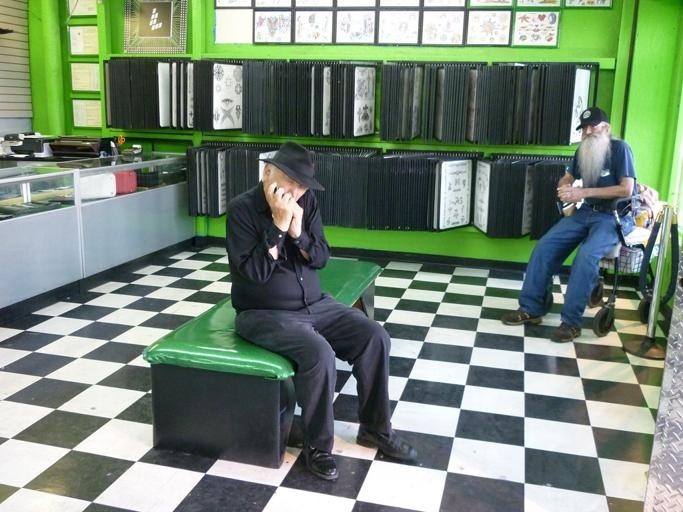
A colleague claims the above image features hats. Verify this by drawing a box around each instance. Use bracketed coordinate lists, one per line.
[575, 107, 608, 131]
[255, 141, 325, 192]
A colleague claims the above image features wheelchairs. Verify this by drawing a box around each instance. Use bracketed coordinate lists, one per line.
[556, 196, 650, 337]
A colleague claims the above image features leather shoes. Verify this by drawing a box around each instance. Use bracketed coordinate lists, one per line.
[356, 423, 417, 461]
[302, 436, 338, 480]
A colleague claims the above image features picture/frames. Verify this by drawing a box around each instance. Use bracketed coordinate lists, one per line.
[123, 1, 187, 56]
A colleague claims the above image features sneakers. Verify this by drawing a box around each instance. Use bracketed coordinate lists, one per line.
[551, 322, 581, 342]
[501, 308, 541, 326]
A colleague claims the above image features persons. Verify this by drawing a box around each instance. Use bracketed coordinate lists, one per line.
[502, 108, 639, 343]
[226, 142, 419, 480]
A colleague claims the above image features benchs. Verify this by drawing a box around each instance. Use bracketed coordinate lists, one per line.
[142, 257, 380, 456]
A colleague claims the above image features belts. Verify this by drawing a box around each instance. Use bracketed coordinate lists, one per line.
[589, 204, 620, 215]
